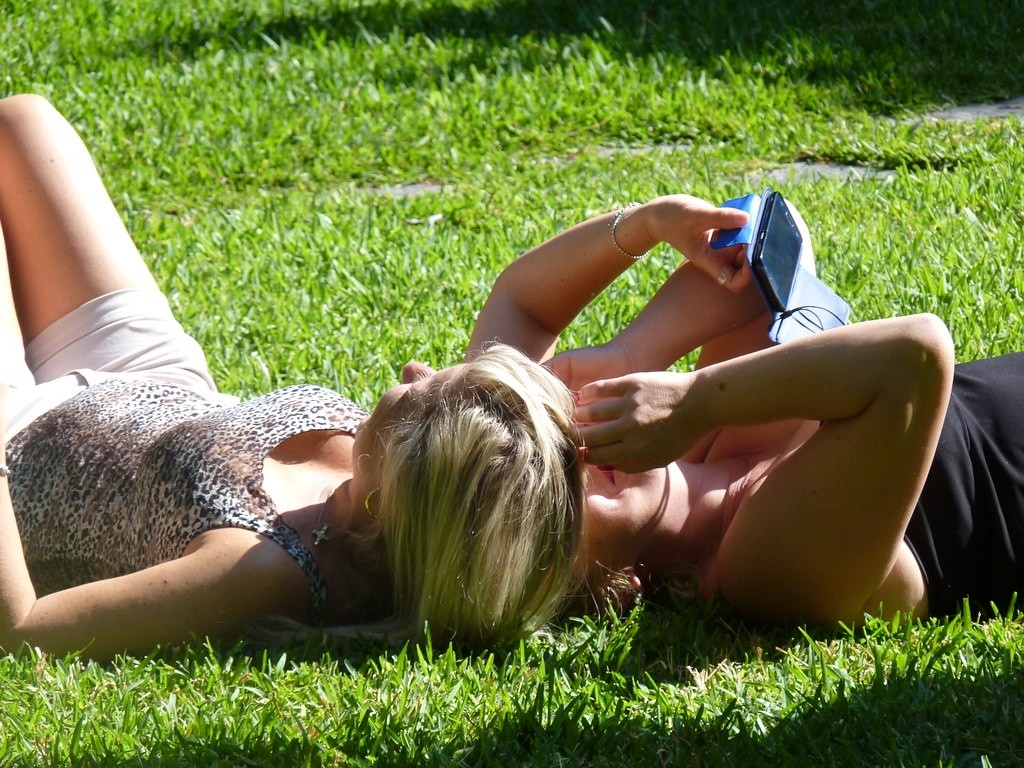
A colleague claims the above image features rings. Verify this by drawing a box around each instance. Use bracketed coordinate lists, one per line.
[718, 273, 727, 287]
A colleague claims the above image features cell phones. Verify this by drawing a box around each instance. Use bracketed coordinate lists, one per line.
[752, 192, 804, 312]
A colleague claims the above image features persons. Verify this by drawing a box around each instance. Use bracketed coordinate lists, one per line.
[0, 93, 754, 665]
[538, 244, 1024, 635]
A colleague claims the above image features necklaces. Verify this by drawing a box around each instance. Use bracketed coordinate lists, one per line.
[313, 503, 330, 545]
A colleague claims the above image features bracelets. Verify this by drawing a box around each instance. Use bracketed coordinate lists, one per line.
[608, 202, 650, 259]
